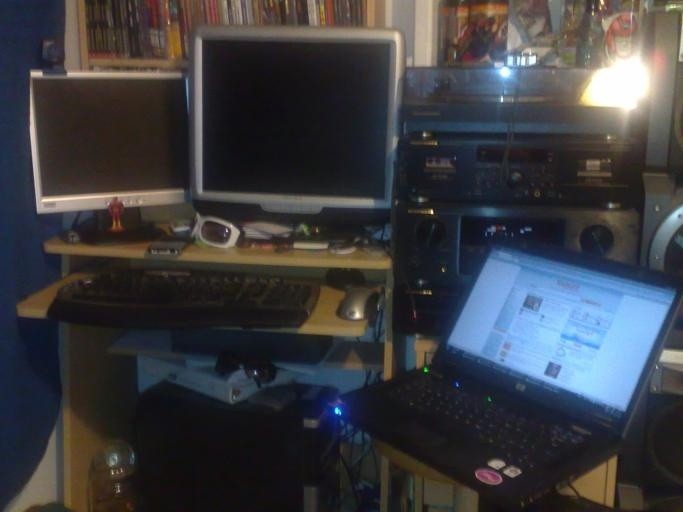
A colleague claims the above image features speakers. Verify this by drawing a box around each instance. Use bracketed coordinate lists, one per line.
[644, 12, 682, 171]
[639, 171, 683, 396]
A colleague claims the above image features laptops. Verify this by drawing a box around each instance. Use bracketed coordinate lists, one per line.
[337, 232, 683, 512]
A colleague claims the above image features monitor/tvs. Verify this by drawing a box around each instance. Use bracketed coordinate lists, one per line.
[190, 24, 404, 245]
[28, 68, 192, 247]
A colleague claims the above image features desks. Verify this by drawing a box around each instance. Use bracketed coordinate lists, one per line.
[11, 236, 398, 512]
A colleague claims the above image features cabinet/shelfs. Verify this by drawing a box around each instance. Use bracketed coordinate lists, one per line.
[75, 0, 377, 74]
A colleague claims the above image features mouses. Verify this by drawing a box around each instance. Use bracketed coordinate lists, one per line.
[335, 284, 377, 322]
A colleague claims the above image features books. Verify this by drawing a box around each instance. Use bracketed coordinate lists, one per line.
[82, 0, 364, 60]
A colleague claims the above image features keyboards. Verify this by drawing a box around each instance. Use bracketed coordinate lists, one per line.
[46, 269, 321, 330]
